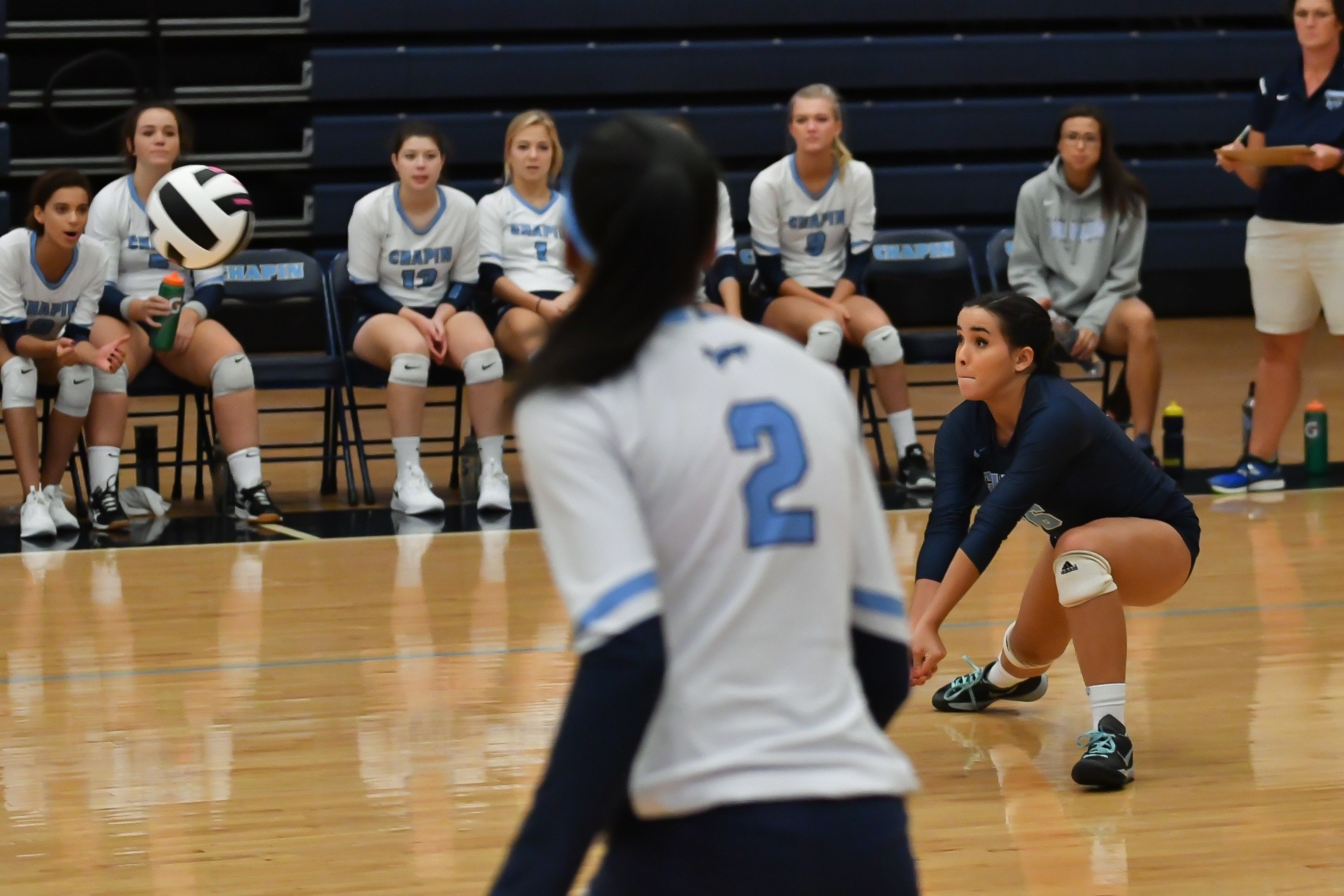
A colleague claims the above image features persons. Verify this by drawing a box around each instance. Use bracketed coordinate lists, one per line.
[0, 0, 1344, 543]
[478, 110, 925, 896]
[908, 291, 1202, 792]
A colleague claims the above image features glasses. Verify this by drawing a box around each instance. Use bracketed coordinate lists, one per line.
[1061, 132, 1100, 148]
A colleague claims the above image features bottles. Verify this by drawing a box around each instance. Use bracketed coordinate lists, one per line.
[1303, 400, 1327, 475]
[1162, 400, 1184, 481]
[149, 270, 186, 352]
[1241, 380, 1256, 455]
[1048, 309, 1104, 378]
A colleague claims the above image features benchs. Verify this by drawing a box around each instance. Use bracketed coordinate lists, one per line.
[298, 0, 1344, 280]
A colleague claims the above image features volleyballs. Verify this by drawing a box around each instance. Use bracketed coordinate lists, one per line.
[146, 165, 256, 270]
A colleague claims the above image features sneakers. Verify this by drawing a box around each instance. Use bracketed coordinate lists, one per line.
[1072, 713, 1135, 787]
[932, 713, 1042, 760]
[1207, 493, 1285, 524]
[87, 474, 132, 530]
[1204, 446, 1285, 494]
[235, 523, 284, 566]
[390, 461, 444, 515]
[88, 532, 133, 577]
[234, 481, 284, 522]
[476, 512, 510, 548]
[21, 483, 57, 538]
[476, 457, 512, 511]
[895, 443, 936, 490]
[45, 530, 79, 566]
[391, 512, 444, 548]
[21, 538, 56, 573]
[43, 485, 80, 529]
[932, 655, 1048, 713]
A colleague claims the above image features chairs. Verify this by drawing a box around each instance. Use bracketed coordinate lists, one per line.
[0, 229, 1125, 514]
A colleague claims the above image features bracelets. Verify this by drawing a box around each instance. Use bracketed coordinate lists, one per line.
[535, 297, 543, 313]
